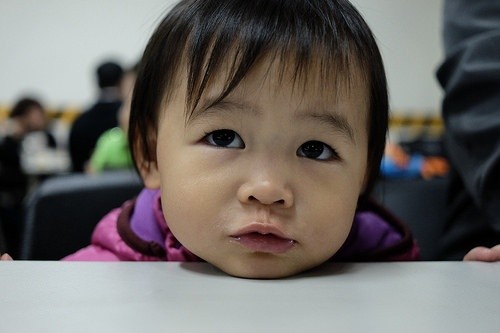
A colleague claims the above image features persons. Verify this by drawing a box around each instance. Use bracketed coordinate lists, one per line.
[0, 2, 500, 279]
[5, 58, 144, 178]
[433, 5, 500, 264]
[382, 114, 447, 186]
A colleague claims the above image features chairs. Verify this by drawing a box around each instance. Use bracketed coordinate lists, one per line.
[24, 170, 447, 264]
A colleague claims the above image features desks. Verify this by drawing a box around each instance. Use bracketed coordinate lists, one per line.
[0, 260, 500, 330]
